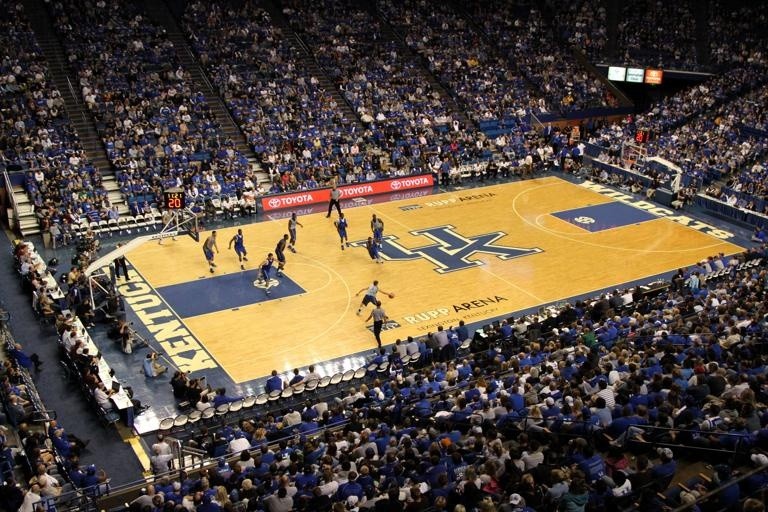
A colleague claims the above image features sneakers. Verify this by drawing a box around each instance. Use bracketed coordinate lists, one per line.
[375, 244, 383, 263]
[326, 214, 330, 218]
[159, 237, 178, 244]
[341, 242, 349, 250]
[243, 257, 248, 261]
[209, 263, 216, 273]
[287, 245, 296, 253]
[276, 266, 284, 277]
[265, 288, 271, 295]
[240, 266, 245, 271]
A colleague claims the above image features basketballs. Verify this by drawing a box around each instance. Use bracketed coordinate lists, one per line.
[388, 292, 395, 299]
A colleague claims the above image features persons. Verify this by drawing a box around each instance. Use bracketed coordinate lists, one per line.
[274, 234, 289, 276]
[258, 253, 284, 296]
[8, 231, 130, 333]
[371, 214, 384, 251]
[366, 301, 388, 347]
[355, 280, 392, 316]
[437, 245, 768, 511]
[334, 213, 349, 250]
[203, 231, 220, 274]
[0, 1, 768, 248]
[366, 237, 384, 264]
[229, 229, 248, 270]
[0, 325, 448, 510]
[288, 213, 303, 253]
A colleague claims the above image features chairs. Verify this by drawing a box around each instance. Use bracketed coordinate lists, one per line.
[557, 2, 766, 228]
[2, 237, 143, 508]
[535, 242, 766, 510]
[144, 315, 536, 509]
[3, 2, 557, 246]
